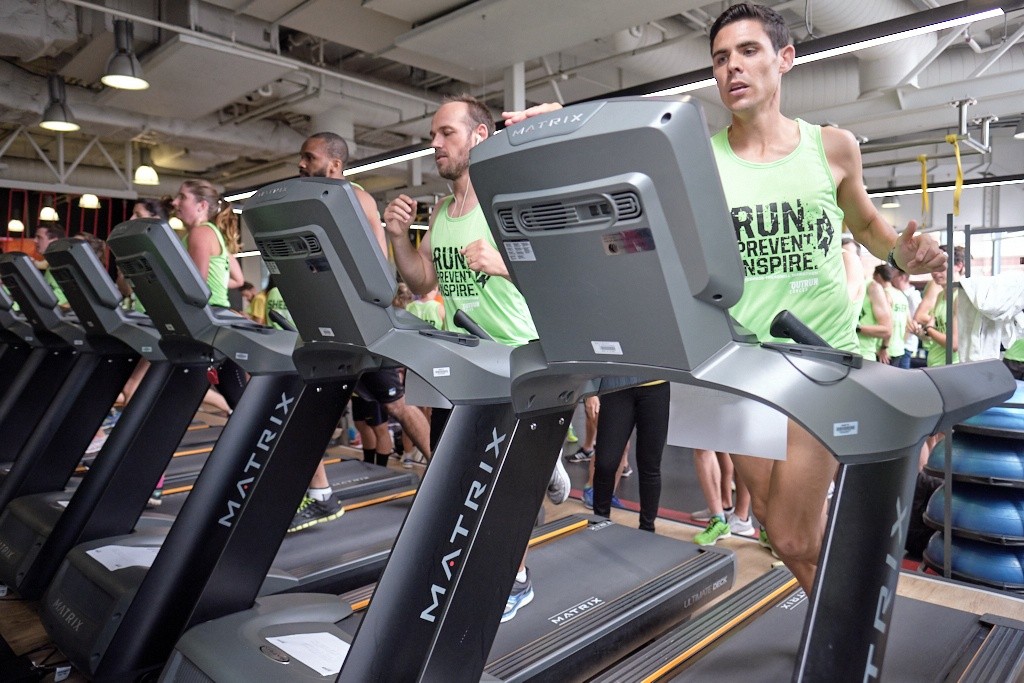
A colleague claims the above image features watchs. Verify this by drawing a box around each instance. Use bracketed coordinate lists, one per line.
[881, 345, 887, 349]
[856, 324, 862, 333]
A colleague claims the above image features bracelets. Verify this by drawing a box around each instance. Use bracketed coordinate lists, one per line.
[925, 326, 934, 331]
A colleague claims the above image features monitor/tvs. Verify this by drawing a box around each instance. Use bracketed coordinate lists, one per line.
[0, 95, 745, 312]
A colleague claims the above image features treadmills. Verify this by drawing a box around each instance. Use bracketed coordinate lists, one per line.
[29, 217, 291, 683]
[1, 247, 86, 481]
[338, 97, 1023, 683]
[1, 241, 162, 592]
[142, 178, 511, 683]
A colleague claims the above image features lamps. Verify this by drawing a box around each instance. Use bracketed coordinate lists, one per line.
[39, 64, 81, 131]
[100, 19, 150, 90]
[79, 193, 101, 208]
[7, 191, 24, 232]
[133, 147, 161, 185]
[882, 179, 901, 209]
[38, 194, 59, 221]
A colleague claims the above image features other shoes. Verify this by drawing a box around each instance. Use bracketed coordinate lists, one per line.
[147, 488, 164, 506]
[350, 437, 363, 448]
[400, 454, 413, 468]
[83, 458, 94, 470]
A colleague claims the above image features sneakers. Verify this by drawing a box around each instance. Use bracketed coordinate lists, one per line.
[727, 514, 760, 539]
[287, 493, 344, 532]
[693, 515, 731, 546]
[546, 448, 571, 505]
[611, 495, 625, 509]
[499, 566, 534, 623]
[691, 506, 735, 521]
[567, 427, 578, 443]
[568, 447, 596, 463]
[581, 484, 594, 509]
[622, 464, 632, 477]
[759, 526, 781, 559]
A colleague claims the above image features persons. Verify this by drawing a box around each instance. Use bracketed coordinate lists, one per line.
[250, 275, 276, 324]
[907, 246, 973, 473]
[285, 132, 430, 530]
[34, 222, 71, 308]
[351, 392, 392, 467]
[688, 449, 755, 547]
[266, 286, 296, 330]
[592, 379, 672, 532]
[503, 0, 949, 601]
[564, 396, 633, 477]
[148, 179, 245, 508]
[392, 282, 411, 459]
[119, 197, 234, 416]
[841, 237, 922, 370]
[75, 233, 111, 454]
[241, 282, 256, 318]
[400, 286, 446, 468]
[386, 92, 571, 622]
